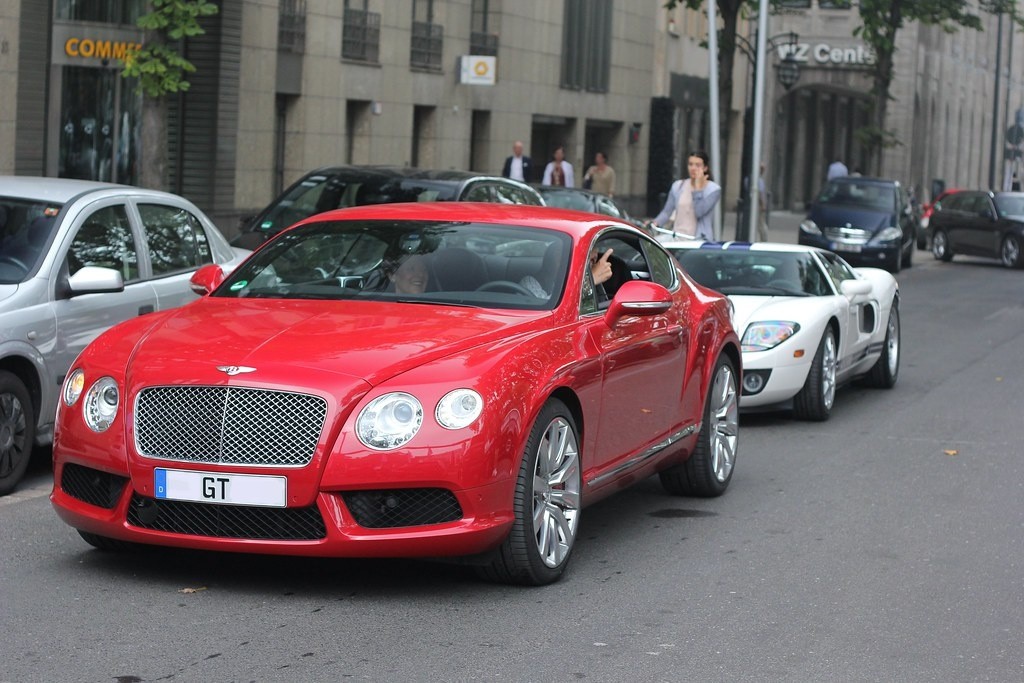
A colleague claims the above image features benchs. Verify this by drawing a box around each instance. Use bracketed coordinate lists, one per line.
[474, 252, 651, 292]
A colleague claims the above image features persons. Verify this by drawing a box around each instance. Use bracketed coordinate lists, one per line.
[516, 240, 614, 300]
[643, 151, 721, 242]
[745, 166, 768, 242]
[383, 252, 429, 293]
[1012, 173, 1020, 191]
[827, 156, 848, 180]
[541, 146, 574, 188]
[851, 168, 862, 176]
[501, 141, 533, 182]
[585, 152, 616, 198]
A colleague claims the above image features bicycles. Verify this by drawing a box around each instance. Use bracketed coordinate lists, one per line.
[644, 222, 702, 241]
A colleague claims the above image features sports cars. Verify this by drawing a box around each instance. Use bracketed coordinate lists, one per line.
[662, 241, 900, 424]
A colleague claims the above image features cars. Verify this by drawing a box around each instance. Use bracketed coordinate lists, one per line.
[226, 163, 547, 288]
[526, 185, 653, 239]
[797, 177, 918, 269]
[918, 188, 1023, 266]
[1, 171, 274, 492]
[50, 202, 746, 586]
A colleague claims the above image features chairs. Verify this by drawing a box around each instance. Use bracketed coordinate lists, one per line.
[424, 248, 489, 292]
[534, 252, 632, 299]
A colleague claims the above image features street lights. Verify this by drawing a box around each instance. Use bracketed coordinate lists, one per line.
[721, 30, 801, 243]
